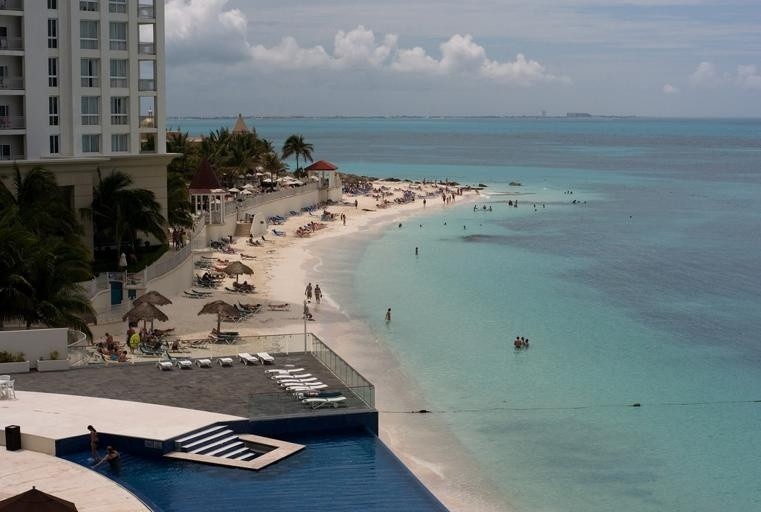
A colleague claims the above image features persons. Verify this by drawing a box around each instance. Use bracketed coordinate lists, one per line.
[91, 322, 179, 362]
[91, 446, 123, 471]
[172, 225, 188, 251]
[513, 336, 531, 350]
[299, 209, 333, 235]
[341, 176, 590, 230]
[304, 282, 314, 300]
[385, 308, 392, 322]
[202, 273, 210, 283]
[88, 426, 101, 461]
[314, 284, 322, 304]
[415, 247, 419, 255]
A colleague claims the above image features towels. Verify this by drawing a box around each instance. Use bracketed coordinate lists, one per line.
[5, 425, 21, 451]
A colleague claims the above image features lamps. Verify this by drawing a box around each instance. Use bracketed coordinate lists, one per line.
[0, 375, 16, 400]
[84, 288, 348, 410]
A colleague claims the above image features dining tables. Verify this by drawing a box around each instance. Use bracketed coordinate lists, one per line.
[0, 351, 69, 373]
